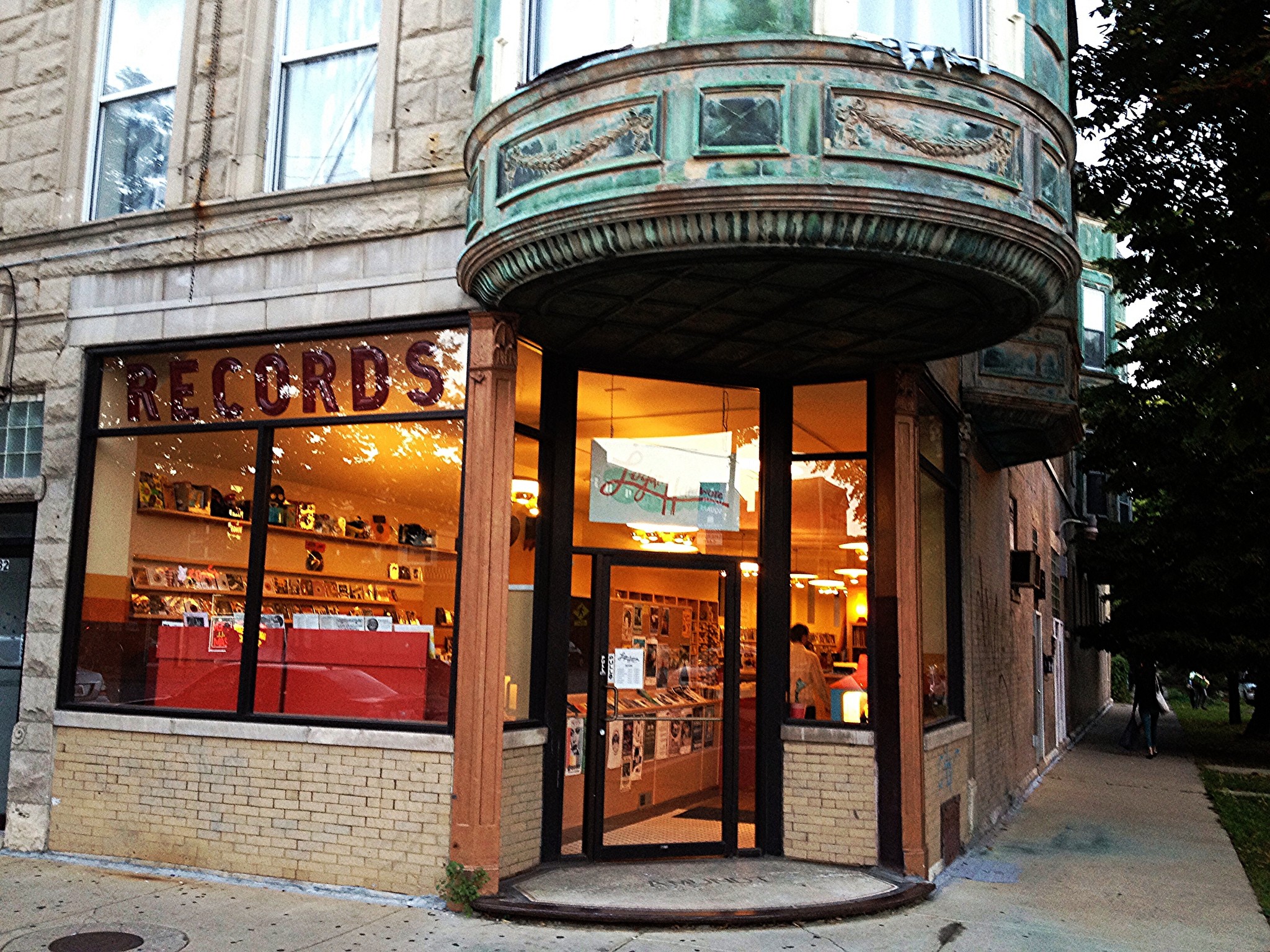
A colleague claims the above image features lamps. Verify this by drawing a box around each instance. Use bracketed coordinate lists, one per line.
[509, 374, 868, 596]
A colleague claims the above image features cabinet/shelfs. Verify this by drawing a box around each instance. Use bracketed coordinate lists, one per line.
[125, 505, 868, 689]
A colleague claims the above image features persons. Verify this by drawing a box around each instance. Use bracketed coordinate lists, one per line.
[804, 641, 815, 652]
[128, 564, 420, 634]
[568, 603, 723, 780]
[784, 623, 832, 720]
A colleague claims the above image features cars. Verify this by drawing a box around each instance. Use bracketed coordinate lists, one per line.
[1238, 671, 1258, 701]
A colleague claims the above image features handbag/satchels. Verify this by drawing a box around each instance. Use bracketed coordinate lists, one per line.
[1120, 715, 1142, 751]
[1154, 673, 1171, 716]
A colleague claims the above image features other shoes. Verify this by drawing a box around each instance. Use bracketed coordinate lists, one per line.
[1149, 751, 1158, 758]
